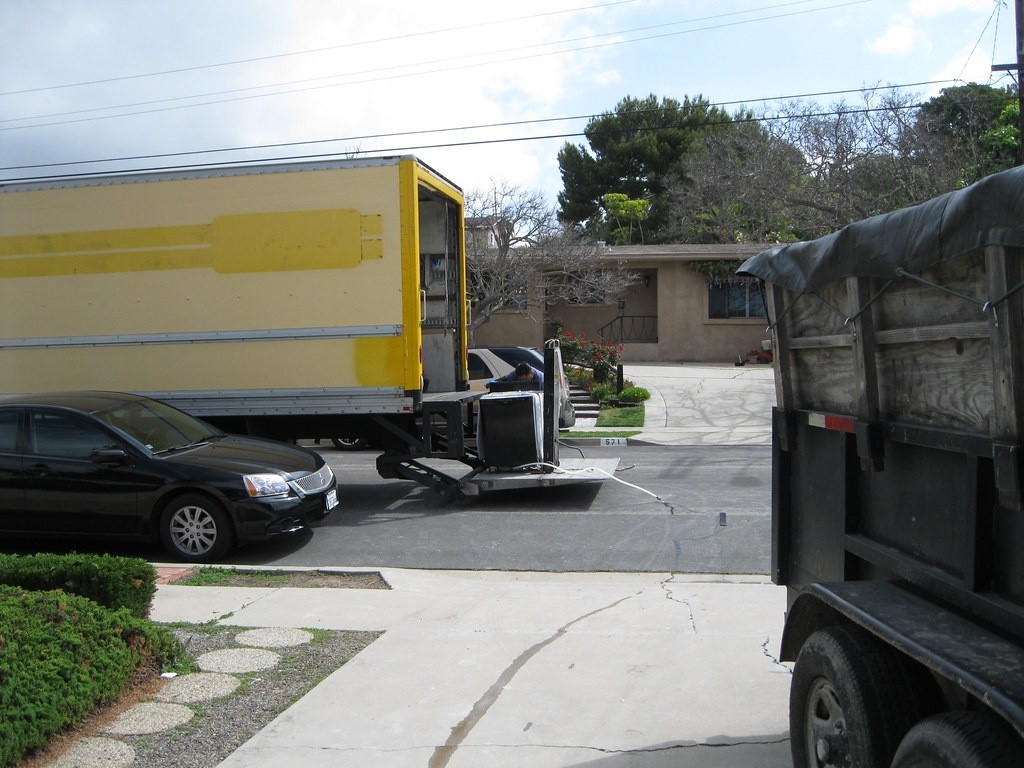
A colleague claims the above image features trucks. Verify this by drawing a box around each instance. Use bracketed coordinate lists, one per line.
[753, 164, 1024, 768]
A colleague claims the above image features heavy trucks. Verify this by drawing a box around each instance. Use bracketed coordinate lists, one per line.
[0, 154, 490, 446]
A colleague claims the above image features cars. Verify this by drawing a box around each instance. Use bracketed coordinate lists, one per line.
[1, 392, 340, 563]
[332, 346, 576, 451]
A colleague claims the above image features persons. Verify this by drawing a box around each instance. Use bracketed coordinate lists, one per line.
[485, 361, 544, 391]
